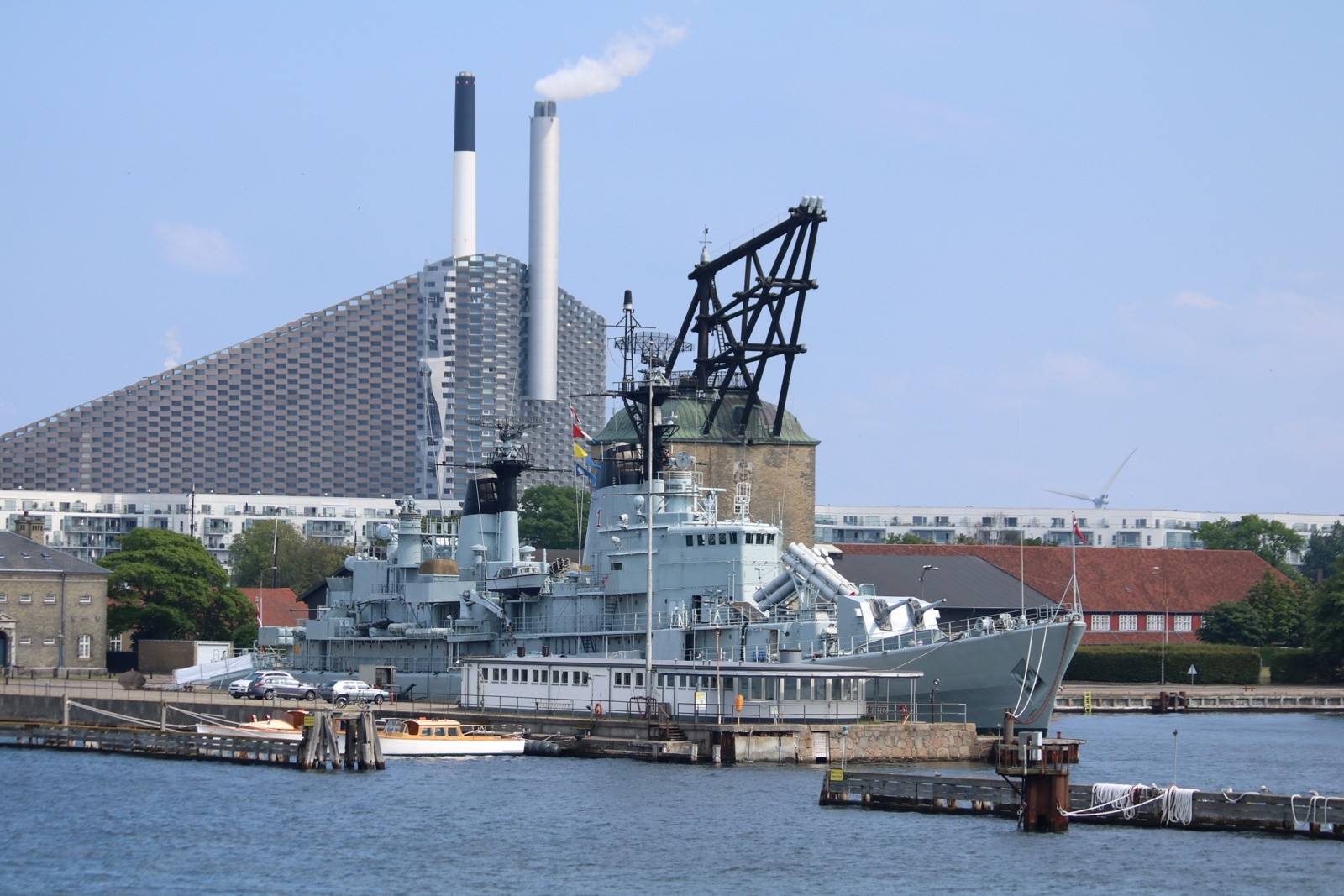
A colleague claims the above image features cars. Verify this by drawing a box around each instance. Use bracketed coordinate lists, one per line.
[318, 680, 392, 707]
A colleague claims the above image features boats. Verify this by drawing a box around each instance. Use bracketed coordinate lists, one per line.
[253, 194, 1087, 740]
[197, 709, 527, 756]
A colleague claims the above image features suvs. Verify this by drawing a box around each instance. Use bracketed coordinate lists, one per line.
[225, 668, 320, 700]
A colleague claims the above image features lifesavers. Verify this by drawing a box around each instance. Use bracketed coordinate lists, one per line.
[594, 703, 603, 719]
[899, 705, 908, 718]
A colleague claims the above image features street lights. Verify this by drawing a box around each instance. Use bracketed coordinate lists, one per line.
[1152, 565, 1166, 685]
[643, 339, 658, 717]
[260, 565, 278, 626]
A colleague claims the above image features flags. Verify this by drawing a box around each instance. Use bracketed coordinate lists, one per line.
[573, 442, 602, 472]
[572, 423, 598, 446]
[570, 406, 583, 425]
[575, 462, 598, 489]
